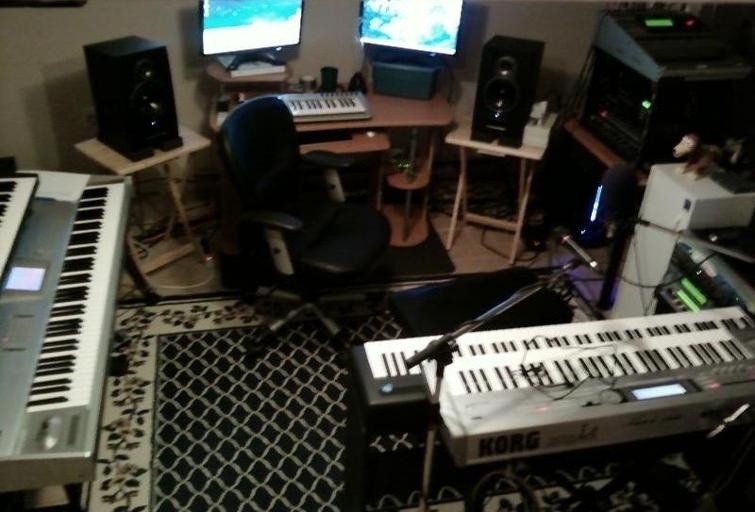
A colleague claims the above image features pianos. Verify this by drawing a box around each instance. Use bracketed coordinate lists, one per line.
[0, 170, 133, 490]
[419, 305, 755, 464]
[352, 320, 614, 418]
[282, 92, 365, 116]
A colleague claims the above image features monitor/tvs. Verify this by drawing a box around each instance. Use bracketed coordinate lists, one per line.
[358, 0, 465, 102]
[195, 0, 305, 73]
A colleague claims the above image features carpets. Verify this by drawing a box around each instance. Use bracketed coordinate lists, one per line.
[0, 268, 726, 512]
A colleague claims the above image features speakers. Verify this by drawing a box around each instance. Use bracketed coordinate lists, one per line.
[470, 33, 545, 148]
[80, 34, 184, 163]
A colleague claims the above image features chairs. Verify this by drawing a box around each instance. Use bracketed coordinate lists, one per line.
[212, 95, 394, 356]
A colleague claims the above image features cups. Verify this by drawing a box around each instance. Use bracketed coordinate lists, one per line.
[320, 66, 337, 92]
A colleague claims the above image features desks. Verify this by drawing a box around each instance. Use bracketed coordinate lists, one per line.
[442, 117, 548, 266]
[539, 112, 650, 313]
[74, 123, 213, 306]
[206, 79, 455, 249]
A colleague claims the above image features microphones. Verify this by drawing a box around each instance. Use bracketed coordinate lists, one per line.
[598, 160, 638, 240]
[549, 224, 605, 275]
